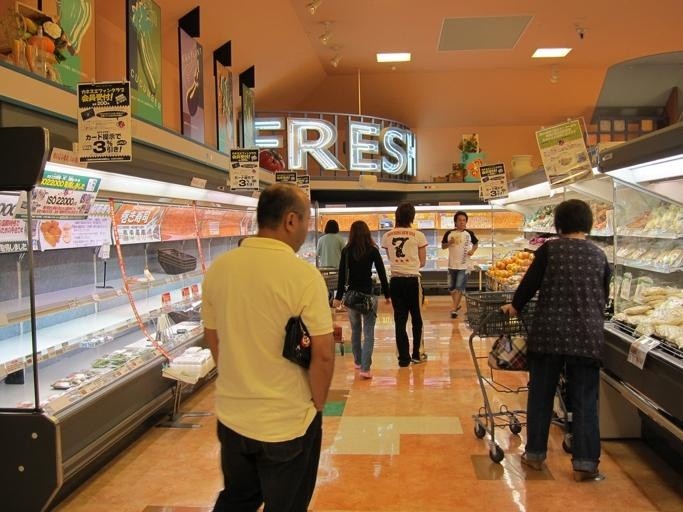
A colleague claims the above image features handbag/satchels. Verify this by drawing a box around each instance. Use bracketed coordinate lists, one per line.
[488, 335, 529, 371]
[343, 288, 375, 314]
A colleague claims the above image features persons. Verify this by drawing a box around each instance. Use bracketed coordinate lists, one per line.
[502, 198, 611, 482]
[316, 220, 347, 311]
[381, 203, 428, 367]
[333, 220, 391, 378]
[441, 210, 478, 318]
[200, 184, 336, 512]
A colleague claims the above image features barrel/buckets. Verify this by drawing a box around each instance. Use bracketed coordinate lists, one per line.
[510, 154, 535, 179]
[460, 152, 488, 182]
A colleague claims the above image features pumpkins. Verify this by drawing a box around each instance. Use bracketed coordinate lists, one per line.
[27, 26, 55, 54]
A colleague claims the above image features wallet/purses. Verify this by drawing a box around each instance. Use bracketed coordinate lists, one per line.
[283, 317, 311, 368]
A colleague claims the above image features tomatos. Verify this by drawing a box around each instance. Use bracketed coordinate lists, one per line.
[259, 148, 285, 175]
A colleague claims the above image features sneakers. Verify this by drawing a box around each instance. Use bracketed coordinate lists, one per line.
[450, 305, 461, 318]
[399, 353, 427, 367]
[575, 471, 598, 481]
[354, 364, 370, 378]
[521, 452, 541, 469]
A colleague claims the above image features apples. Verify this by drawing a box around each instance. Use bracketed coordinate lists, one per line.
[488, 251, 535, 284]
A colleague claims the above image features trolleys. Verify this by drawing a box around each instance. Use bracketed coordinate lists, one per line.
[463, 290, 575, 463]
[320, 267, 346, 356]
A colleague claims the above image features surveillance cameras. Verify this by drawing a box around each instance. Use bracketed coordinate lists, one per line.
[579, 31, 585, 41]
